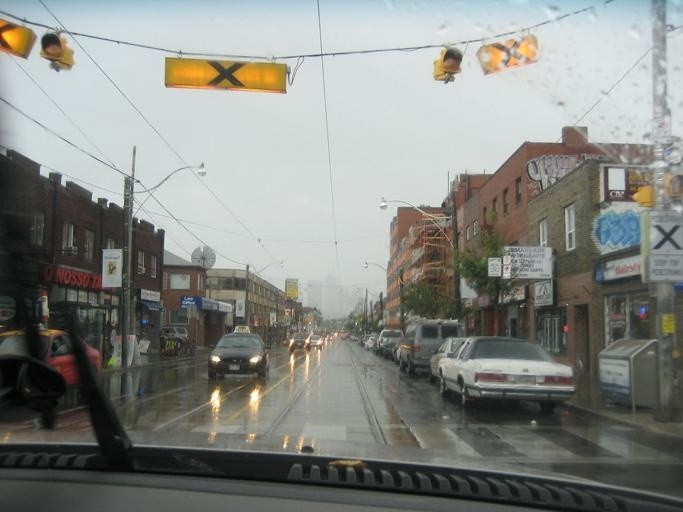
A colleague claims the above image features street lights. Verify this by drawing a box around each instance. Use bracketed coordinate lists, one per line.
[364, 261, 404, 329]
[349, 284, 383, 329]
[242, 256, 286, 324]
[124, 143, 207, 335]
[378, 189, 461, 320]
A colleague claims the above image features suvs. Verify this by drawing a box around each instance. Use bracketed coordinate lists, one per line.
[289, 332, 310, 352]
[397, 319, 467, 376]
[160, 327, 191, 344]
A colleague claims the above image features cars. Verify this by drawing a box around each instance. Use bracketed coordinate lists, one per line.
[435, 335, 575, 415]
[428, 337, 467, 383]
[310, 330, 338, 350]
[0, 322, 101, 388]
[206, 325, 271, 379]
[338, 329, 403, 365]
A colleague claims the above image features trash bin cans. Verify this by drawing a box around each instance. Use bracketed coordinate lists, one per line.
[597, 339, 658, 407]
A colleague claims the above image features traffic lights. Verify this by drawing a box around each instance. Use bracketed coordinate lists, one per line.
[39, 30, 68, 63]
[437, 45, 463, 79]
